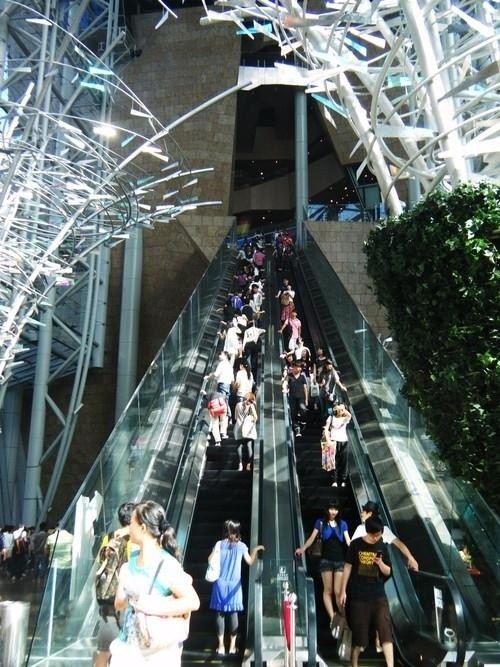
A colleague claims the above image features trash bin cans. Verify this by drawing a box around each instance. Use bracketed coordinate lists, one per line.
[1, 600, 30, 667]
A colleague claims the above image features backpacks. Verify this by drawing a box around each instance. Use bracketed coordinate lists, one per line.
[95, 531, 128, 622]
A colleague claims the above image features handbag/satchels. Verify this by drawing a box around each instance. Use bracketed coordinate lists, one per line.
[209, 392, 226, 416]
[321, 441, 337, 472]
[241, 405, 257, 439]
[284, 317, 293, 342]
[313, 519, 323, 559]
[205, 542, 221, 581]
[339, 602, 353, 662]
[329, 599, 347, 640]
[135, 557, 190, 649]
[281, 294, 289, 305]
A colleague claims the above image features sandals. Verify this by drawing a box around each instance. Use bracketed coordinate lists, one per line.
[216, 648, 225, 654]
[229, 647, 238, 654]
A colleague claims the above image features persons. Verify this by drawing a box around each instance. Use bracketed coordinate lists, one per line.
[213, 232, 266, 333]
[242, 321, 266, 385]
[279, 284, 296, 320]
[313, 346, 341, 409]
[234, 392, 258, 472]
[318, 360, 348, 425]
[93, 501, 136, 666]
[203, 350, 234, 404]
[233, 358, 256, 403]
[207, 519, 264, 654]
[200, 390, 232, 447]
[325, 404, 351, 487]
[272, 232, 295, 266]
[293, 500, 351, 628]
[350, 499, 419, 572]
[275, 277, 297, 299]
[216, 321, 243, 371]
[280, 337, 311, 361]
[0, 523, 47, 586]
[340, 517, 394, 667]
[277, 309, 302, 350]
[282, 363, 309, 438]
[458, 544, 474, 572]
[108, 501, 201, 666]
[297, 348, 313, 401]
[282, 354, 296, 376]
[45, 528, 74, 626]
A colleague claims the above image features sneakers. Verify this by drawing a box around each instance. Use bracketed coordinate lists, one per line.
[314, 406, 319, 410]
[332, 482, 337, 488]
[238, 463, 243, 471]
[341, 482, 346, 488]
[215, 442, 220, 447]
[245, 464, 252, 471]
[221, 433, 229, 438]
[300, 419, 307, 424]
[296, 427, 301, 437]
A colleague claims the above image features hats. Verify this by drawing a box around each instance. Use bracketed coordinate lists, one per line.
[326, 359, 332, 364]
[362, 502, 381, 515]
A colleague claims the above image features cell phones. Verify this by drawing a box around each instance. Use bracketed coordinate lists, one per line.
[373, 551, 382, 566]
